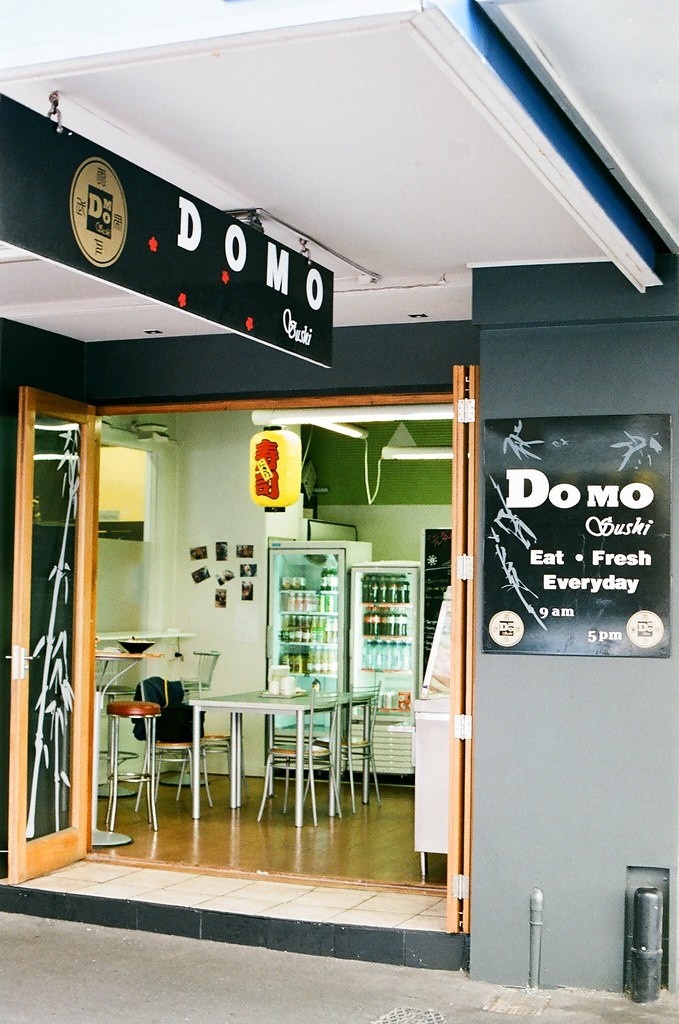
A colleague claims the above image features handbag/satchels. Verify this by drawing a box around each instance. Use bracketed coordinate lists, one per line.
[132, 703, 206, 742]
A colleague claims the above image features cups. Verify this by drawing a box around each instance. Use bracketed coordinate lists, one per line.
[281, 677, 296, 695]
[272, 665, 290, 680]
[268, 680, 279, 695]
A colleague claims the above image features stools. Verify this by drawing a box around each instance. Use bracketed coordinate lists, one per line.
[97, 684, 146, 797]
[156, 650, 222, 786]
[104, 700, 158, 832]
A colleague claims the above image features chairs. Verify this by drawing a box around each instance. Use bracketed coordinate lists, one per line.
[310, 682, 385, 813]
[258, 689, 345, 827]
[178, 678, 253, 800]
[134, 680, 214, 811]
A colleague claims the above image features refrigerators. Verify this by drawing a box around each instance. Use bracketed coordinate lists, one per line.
[348, 561, 424, 774]
[266, 540, 372, 771]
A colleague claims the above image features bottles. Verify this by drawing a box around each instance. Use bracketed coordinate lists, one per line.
[279, 593, 336, 612]
[364, 574, 408, 603]
[279, 646, 336, 674]
[312, 676, 321, 692]
[281, 566, 338, 590]
[366, 640, 411, 671]
[365, 607, 407, 636]
[282, 615, 335, 643]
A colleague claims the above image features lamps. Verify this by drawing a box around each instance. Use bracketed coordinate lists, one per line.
[252, 404, 453, 441]
[381, 444, 455, 461]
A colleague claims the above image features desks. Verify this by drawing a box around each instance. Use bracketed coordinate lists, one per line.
[187, 693, 375, 826]
[91, 653, 166, 848]
[95, 630, 193, 648]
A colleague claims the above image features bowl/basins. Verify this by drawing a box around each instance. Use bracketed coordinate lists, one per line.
[117, 640, 155, 654]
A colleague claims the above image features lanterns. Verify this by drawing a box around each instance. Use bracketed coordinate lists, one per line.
[249, 424, 302, 512]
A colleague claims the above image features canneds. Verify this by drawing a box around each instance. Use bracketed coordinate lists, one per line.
[279, 614, 311, 642]
[378, 692, 398, 709]
[281, 576, 306, 590]
